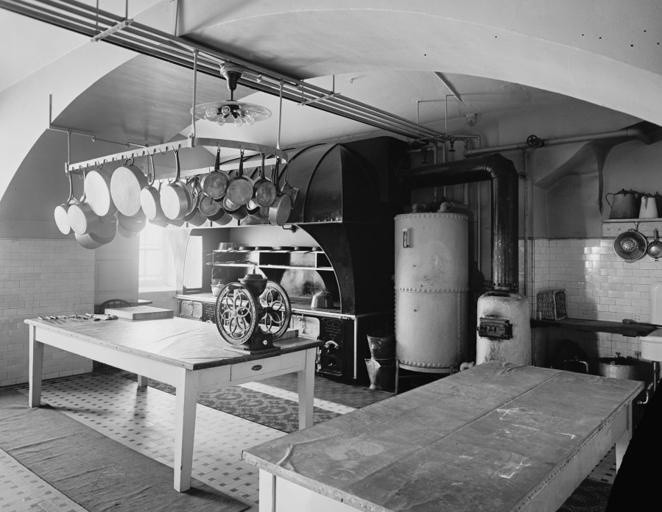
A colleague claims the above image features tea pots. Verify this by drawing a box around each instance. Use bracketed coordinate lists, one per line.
[309, 286, 334, 308]
[605, 188, 661, 218]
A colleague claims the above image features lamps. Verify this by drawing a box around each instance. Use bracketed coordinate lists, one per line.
[189, 63, 271, 126]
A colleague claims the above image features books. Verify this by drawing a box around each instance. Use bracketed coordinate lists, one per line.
[105, 305, 174, 320]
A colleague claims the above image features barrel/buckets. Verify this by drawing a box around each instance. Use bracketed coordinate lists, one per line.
[394, 212, 471, 374]
[597, 355, 644, 380]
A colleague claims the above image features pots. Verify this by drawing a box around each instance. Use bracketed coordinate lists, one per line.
[52, 143, 300, 251]
[209, 281, 226, 296]
[613, 223, 648, 262]
[646, 229, 661, 259]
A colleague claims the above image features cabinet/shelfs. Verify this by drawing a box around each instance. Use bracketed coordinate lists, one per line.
[209, 250, 334, 272]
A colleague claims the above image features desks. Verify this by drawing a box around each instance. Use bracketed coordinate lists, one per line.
[241, 358, 646, 512]
[25, 315, 322, 491]
